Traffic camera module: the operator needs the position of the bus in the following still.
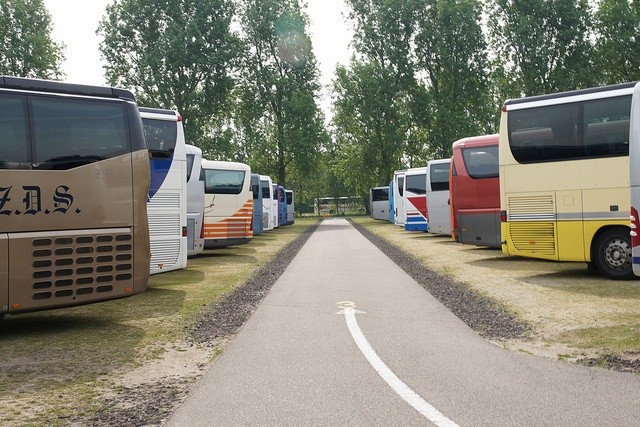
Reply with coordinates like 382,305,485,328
402,166,427,233
314,196,367,217
426,157,451,238
183,142,205,257
259,175,274,231
250,173,262,237
450,132,502,252
278,185,287,225
272,183,280,229
285,189,294,225
0,75,152,318
201,158,253,251
369,186,390,220
389,180,394,224
393,170,405,228
497,80,638,280
629,81,639,280
137,107,189,278
